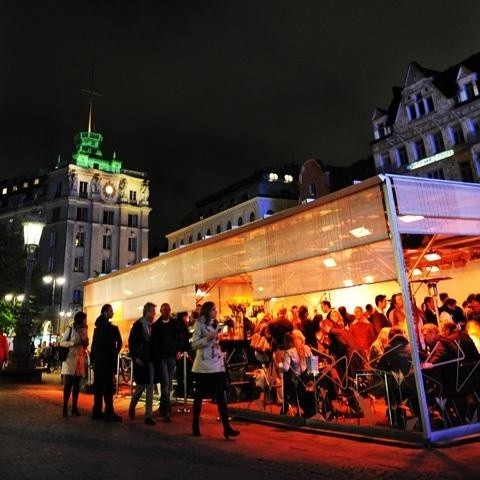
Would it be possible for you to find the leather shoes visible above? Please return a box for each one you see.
[92,409,172,425]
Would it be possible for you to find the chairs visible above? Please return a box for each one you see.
[219,342,480,433]
[83,348,135,400]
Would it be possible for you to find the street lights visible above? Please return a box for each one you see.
[1,216,48,384]
[42,274,66,373]
[5,294,25,323]
[59,310,72,334]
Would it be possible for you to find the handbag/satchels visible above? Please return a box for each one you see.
[57,342,71,362]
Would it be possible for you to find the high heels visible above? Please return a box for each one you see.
[223,427,240,440]
[192,422,201,436]
[63,407,68,417]
[71,407,81,416]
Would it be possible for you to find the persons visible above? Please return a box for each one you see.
[90,305,123,424]
[0,327,9,377]
[59,311,90,418]
